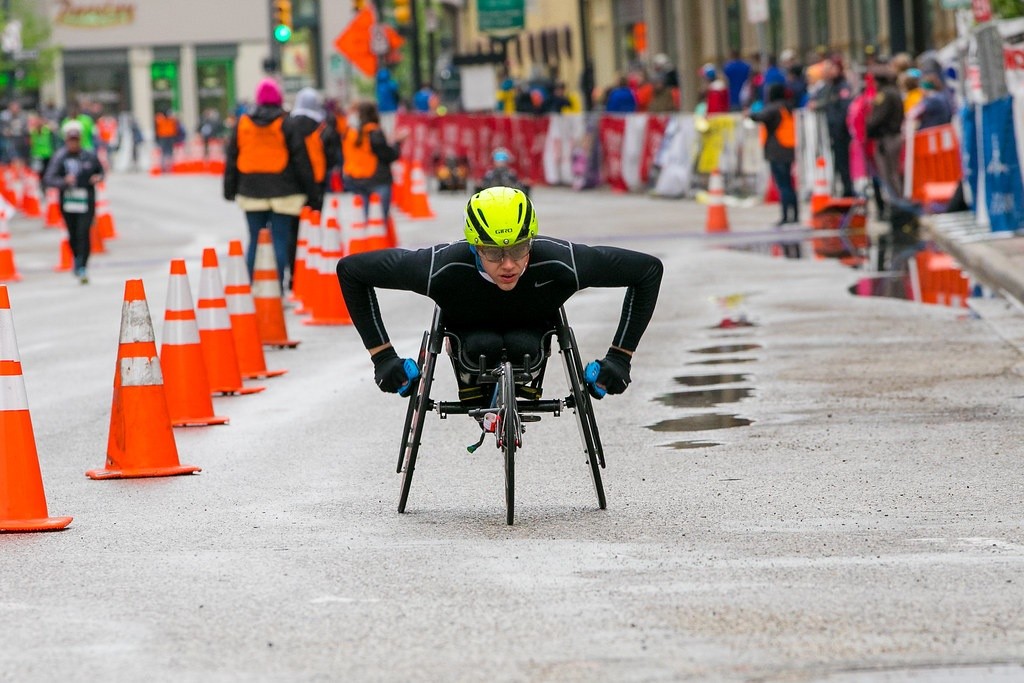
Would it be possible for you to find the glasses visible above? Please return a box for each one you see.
[479,241,531,262]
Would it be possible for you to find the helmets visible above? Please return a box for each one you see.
[463,185,538,247]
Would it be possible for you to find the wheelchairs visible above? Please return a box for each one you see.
[396,287,615,525]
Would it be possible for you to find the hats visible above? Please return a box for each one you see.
[256,78,281,106]
[66,129,80,140]
[290,88,327,123]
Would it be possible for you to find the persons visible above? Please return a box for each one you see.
[224,80,324,308]
[43,128,104,283]
[337,186,664,394]
[1,43,971,290]
[743,83,801,226]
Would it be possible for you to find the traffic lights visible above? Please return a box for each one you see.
[273,0,294,44]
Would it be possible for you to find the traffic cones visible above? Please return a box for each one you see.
[86,277,204,481]
[149,136,228,176]
[704,168,730,233]
[0,283,76,534]
[810,157,831,212]
[0,208,22,282]
[222,240,285,380]
[289,154,434,327]
[194,248,266,396]
[158,259,230,428]
[251,227,302,348]
[0,147,116,273]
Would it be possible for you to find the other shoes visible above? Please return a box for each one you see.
[280,294,298,308]
[79,266,88,284]
[778,219,798,224]
